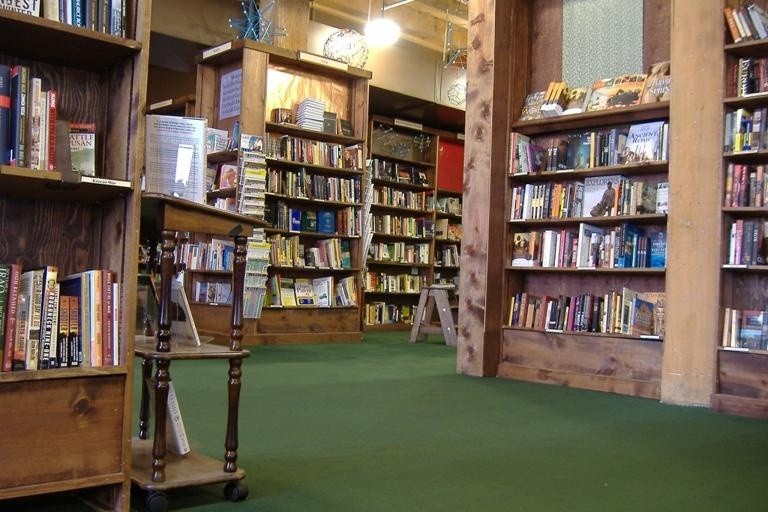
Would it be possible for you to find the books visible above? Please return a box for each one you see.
[517,62,671,121]
[509,122,669,174]
[245,229,359,318]
[150,279,199,348]
[722,0,768,353]
[0,263,120,368]
[204,127,363,237]
[0,0,126,38]
[0,64,95,177]
[273,98,353,136]
[361,159,461,326]
[173,238,232,305]
[511,223,666,269]
[144,374,191,457]
[508,286,665,340]
[509,175,669,220]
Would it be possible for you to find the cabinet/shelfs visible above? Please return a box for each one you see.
[493,35,767,420]
[132,188,271,491]
[0,0,152,511]
